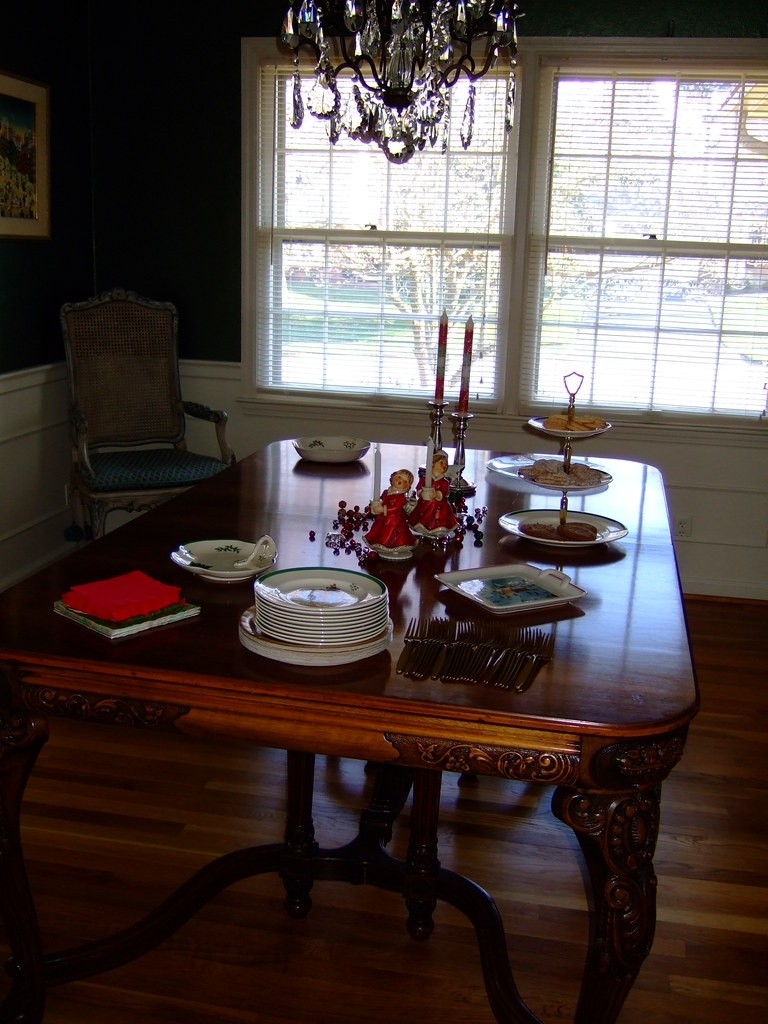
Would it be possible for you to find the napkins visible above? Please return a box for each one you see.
[60,571,180,622]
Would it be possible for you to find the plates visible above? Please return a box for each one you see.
[169,534,278,582]
[291,436,371,463]
[239,567,393,667]
[486,453,598,477]
[433,563,588,613]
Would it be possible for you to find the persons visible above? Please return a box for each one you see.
[363,469,417,555]
[408,449,459,538]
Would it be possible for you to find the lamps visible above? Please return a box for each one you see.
[277,0,518,163]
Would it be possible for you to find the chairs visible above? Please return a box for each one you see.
[61,289,239,542]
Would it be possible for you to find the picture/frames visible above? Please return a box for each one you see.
[0,74,51,239]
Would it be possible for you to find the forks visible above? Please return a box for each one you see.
[395,617,556,694]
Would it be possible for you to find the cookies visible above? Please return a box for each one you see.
[521,522,598,542]
[539,415,606,432]
[518,458,603,487]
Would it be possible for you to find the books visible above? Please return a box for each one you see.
[52,600,201,638]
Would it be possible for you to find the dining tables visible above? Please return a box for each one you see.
[1,434,703,1024]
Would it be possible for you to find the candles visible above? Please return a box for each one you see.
[374,448,380,502]
[424,436,434,488]
[435,307,448,403]
[458,315,474,414]
